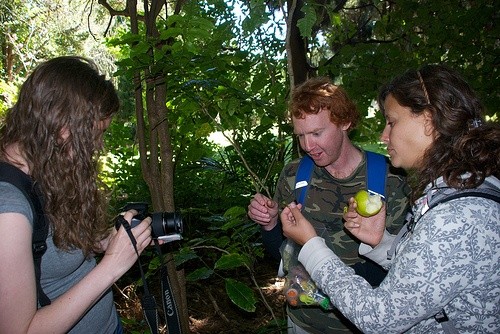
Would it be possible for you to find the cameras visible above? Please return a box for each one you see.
[116,202,184,240]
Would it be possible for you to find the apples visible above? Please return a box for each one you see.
[354,190,383,217]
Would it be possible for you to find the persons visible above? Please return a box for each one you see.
[279,63,500,334]
[0,55,164,334]
[246,76,416,334]
